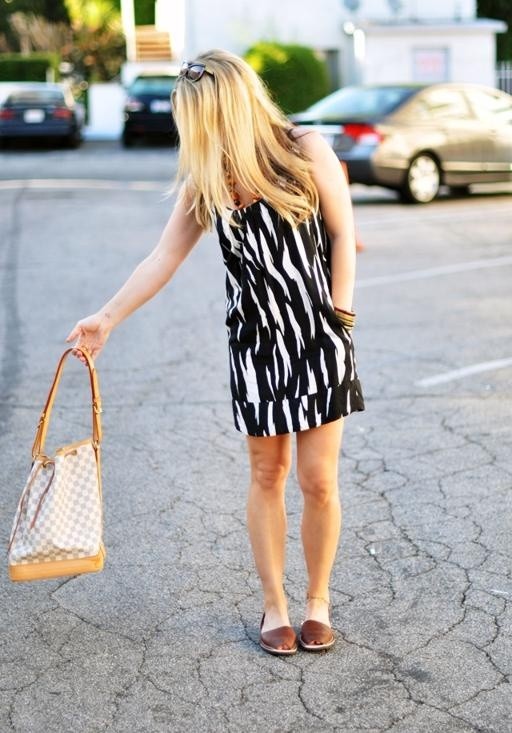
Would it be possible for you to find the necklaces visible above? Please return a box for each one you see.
[221,159,262,209]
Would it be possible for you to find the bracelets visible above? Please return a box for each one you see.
[335,307,356,334]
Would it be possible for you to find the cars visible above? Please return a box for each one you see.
[1,87,85,147]
[121,71,179,145]
[287,82,512,203]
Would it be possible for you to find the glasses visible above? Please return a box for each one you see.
[178,59,215,82]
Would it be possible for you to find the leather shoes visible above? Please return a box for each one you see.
[298,599,335,650]
[259,610,299,655]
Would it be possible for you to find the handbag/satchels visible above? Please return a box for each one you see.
[6,437,107,581]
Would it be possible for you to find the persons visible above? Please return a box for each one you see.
[66,49,364,655]
